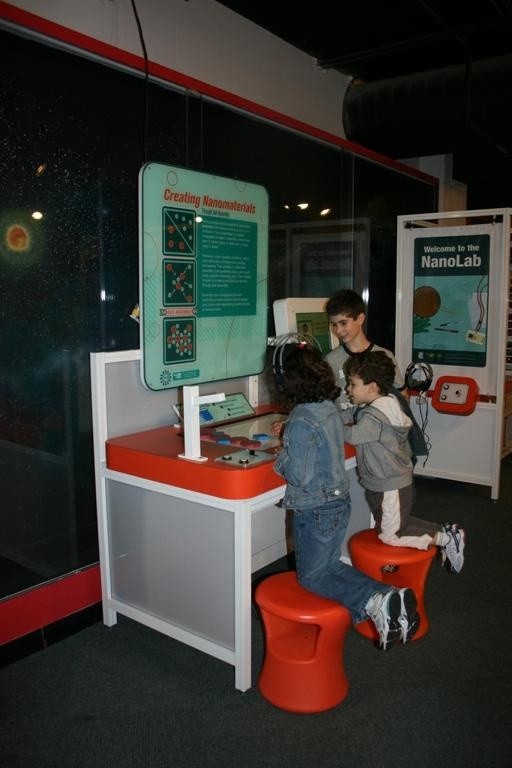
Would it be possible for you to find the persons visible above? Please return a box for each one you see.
[261,342,422,652]
[321,288,411,411]
[337,351,466,574]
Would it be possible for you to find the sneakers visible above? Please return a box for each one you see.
[441,522,460,577]
[367,590,402,652]
[443,529,465,575]
[396,586,421,649]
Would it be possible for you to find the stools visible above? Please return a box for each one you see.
[255,527,438,716]
